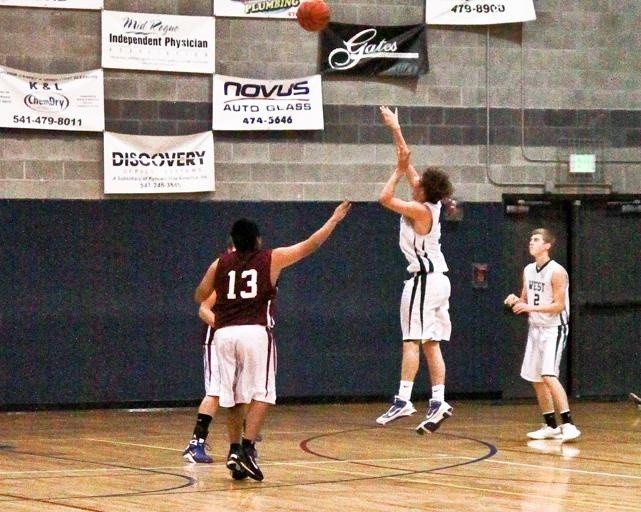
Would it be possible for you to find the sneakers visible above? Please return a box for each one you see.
[375,394,418,425]
[526,423,563,440]
[415,398,454,435]
[560,423,581,443]
[226,445,265,481]
[562,444,580,459]
[527,440,562,457]
[182,434,214,464]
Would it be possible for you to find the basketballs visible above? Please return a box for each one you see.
[297,0,330,32]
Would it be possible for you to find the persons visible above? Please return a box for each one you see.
[501,226,581,445]
[374,101,458,437]
[189,193,354,484]
[180,235,262,465]
